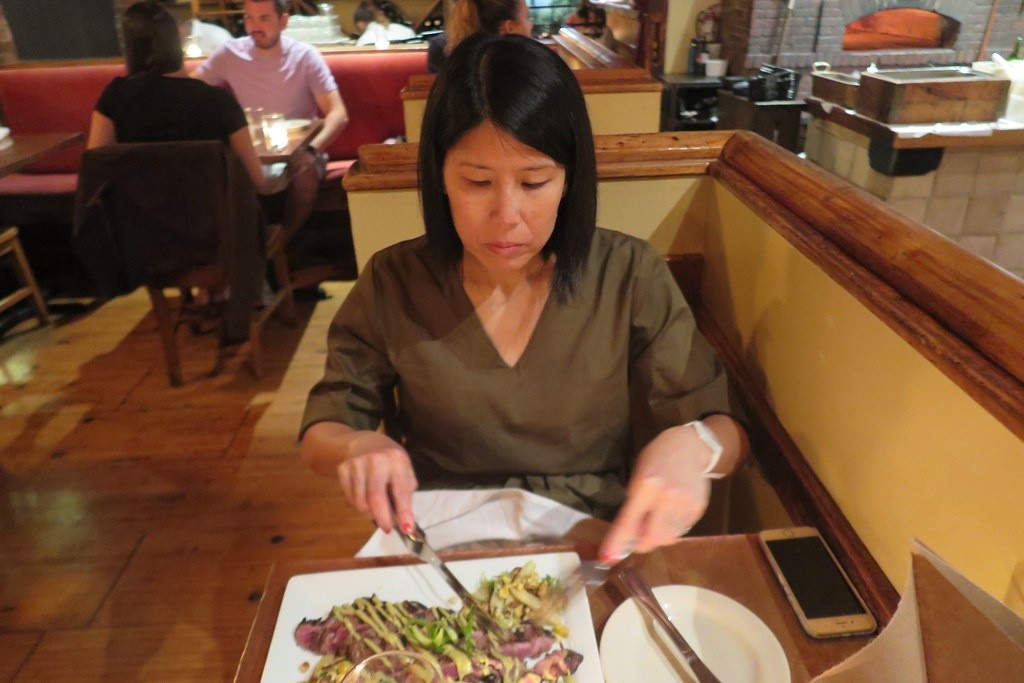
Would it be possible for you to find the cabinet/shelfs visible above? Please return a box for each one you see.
[658,73,726,132]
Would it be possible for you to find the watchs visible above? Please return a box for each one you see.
[304,144,317,155]
[685,420,726,479]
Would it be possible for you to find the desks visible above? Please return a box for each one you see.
[234,533,882,683]
[0,131,84,180]
[255,119,325,334]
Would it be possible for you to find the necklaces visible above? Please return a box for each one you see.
[473,265,545,311]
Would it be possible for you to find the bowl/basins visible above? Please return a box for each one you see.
[705,60,728,77]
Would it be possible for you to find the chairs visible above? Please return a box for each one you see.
[66,140,297,388]
[0,225,52,328]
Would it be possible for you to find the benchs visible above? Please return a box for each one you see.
[0,43,432,269]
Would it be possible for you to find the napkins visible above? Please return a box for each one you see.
[812,539,1023,682]
[355,488,613,558]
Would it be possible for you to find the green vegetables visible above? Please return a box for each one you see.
[405,573,560,651]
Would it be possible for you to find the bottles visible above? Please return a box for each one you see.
[1006,39,1024,61]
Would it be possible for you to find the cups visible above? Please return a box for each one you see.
[261,112,289,151]
[244,106,264,148]
[341,649,442,683]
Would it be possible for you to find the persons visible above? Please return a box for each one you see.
[474,0,534,35]
[85,1,349,332]
[354,4,416,44]
[565,0,613,49]
[297,36,756,564]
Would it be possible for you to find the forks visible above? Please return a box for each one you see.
[534,538,637,623]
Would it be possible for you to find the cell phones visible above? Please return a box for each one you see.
[758,526,877,639]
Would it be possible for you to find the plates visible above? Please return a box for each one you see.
[599,584,792,683]
[286,119,312,131]
[259,551,605,683]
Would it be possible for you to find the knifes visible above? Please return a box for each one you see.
[616,565,722,683]
[389,494,505,636]
